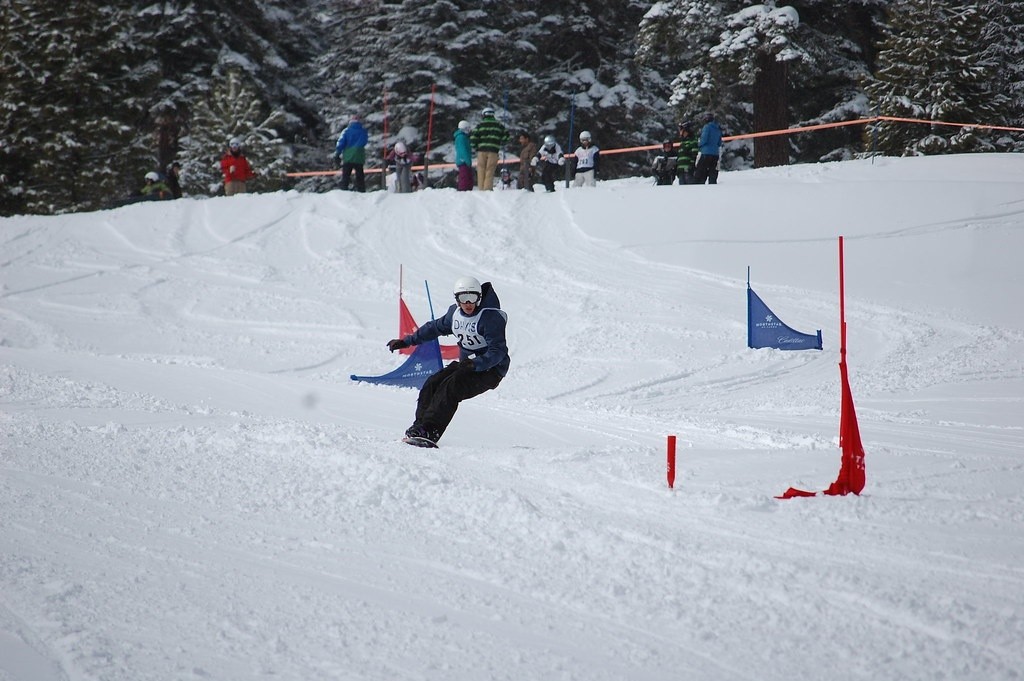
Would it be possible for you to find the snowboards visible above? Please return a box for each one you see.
[401,437,439,448]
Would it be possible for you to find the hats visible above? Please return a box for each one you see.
[679,122,692,132]
[701,112,713,121]
[500,169,510,178]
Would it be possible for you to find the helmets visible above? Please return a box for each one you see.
[453,276,482,307]
[482,107,495,117]
[144,171,159,183]
[580,131,591,143]
[395,142,407,158]
[458,120,470,134]
[545,135,556,147]
[230,138,239,148]
[663,139,673,148]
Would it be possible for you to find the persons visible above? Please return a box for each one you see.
[386,276,510,448]
[126,161,182,201]
[570,131,601,188]
[220,138,257,197]
[497,168,517,190]
[385,142,425,193]
[453,120,474,191]
[530,135,565,193]
[651,112,723,185]
[470,107,509,191]
[335,113,369,193]
[516,132,540,192]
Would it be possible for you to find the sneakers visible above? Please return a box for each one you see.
[405,424,441,443]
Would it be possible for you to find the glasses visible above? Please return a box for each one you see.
[455,291,481,304]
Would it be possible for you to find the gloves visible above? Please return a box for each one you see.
[457,358,476,373]
[387,339,411,353]
